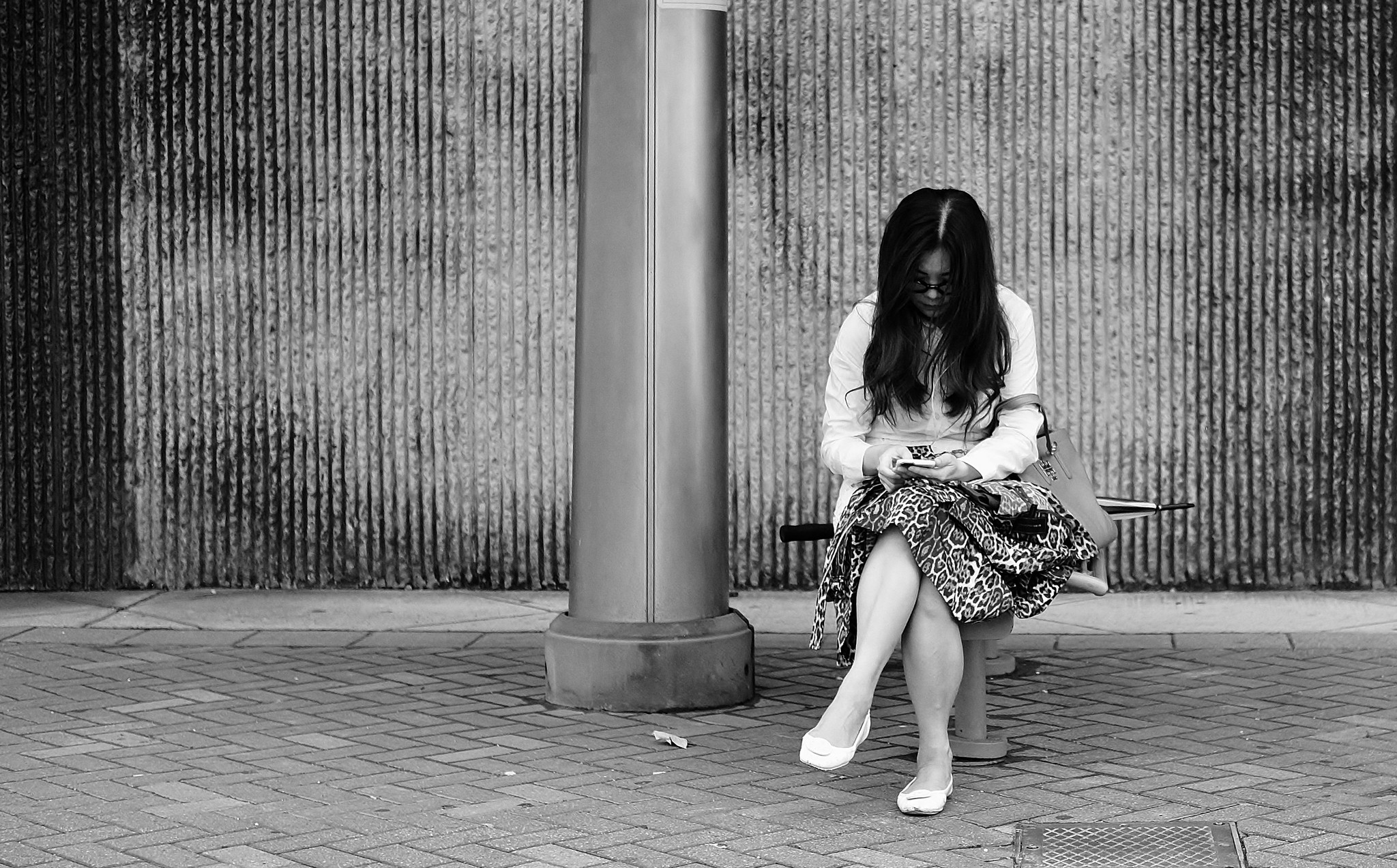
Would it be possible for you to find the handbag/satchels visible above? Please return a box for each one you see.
[997,393,1120,552]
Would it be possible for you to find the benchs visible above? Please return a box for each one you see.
[949,547,1110,758]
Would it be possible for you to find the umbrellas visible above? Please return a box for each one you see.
[780,497,1195,543]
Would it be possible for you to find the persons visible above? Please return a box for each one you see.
[798,185,1100,817]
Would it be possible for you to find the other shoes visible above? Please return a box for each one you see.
[896,772,954,817]
[799,709,872,771]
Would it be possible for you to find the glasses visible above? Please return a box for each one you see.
[909,278,957,295]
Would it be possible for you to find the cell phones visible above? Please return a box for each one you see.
[895,458,935,469]
